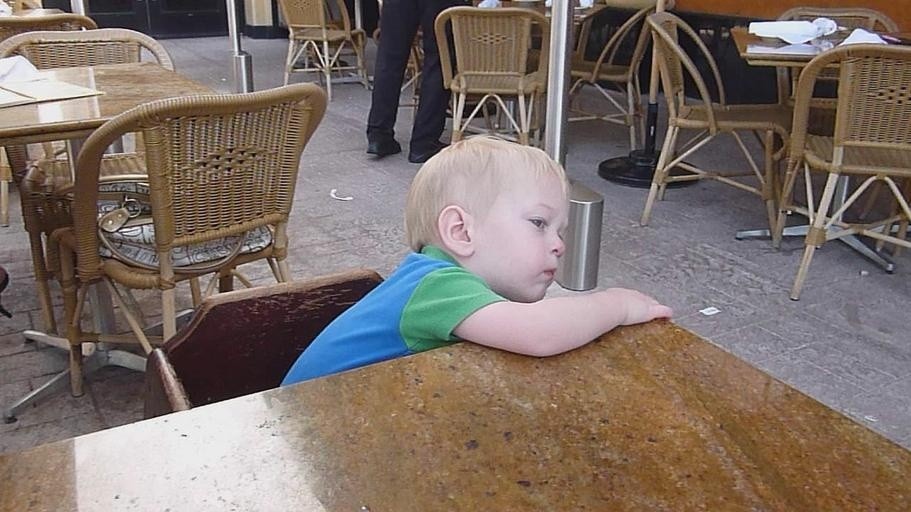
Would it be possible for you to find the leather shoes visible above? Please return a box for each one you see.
[366,139,401,155]
[409,141,449,163]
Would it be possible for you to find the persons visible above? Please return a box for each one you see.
[365,0,473,165]
[280,133,673,387]
